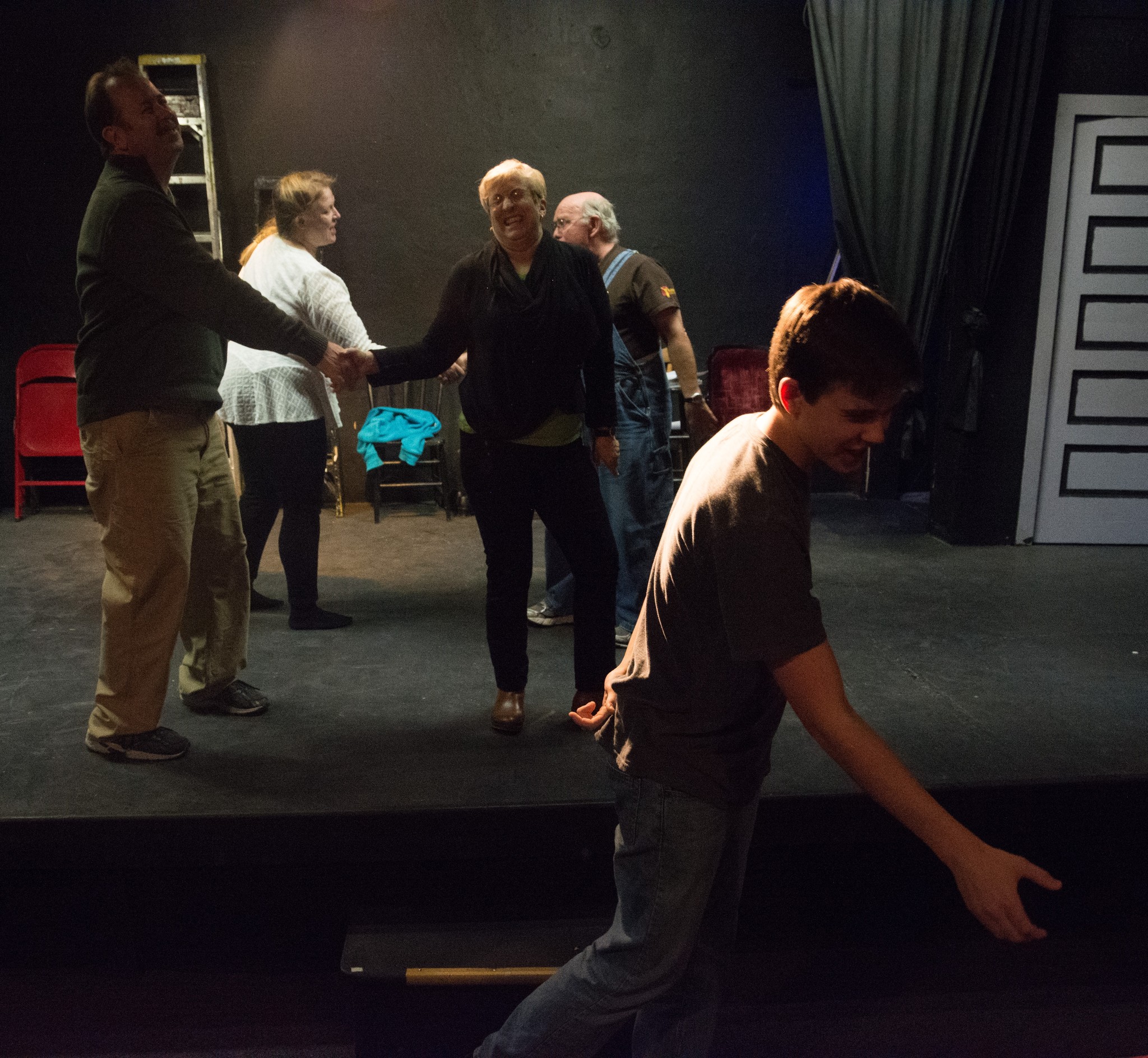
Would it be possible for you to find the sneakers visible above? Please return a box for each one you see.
[288,604,353,629]
[527,599,574,626]
[85,726,192,762]
[614,626,633,648]
[250,590,284,609]
[210,679,269,715]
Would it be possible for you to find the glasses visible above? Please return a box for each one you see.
[486,188,534,207]
[553,216,587,229]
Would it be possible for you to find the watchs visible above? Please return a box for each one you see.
[684,392,704,406]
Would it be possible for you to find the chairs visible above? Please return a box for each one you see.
[316,372,343,518]
[363,374,452,525]
[12,344,84,523]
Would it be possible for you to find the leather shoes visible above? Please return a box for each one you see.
[490,689,525,733]
[571,690,604,718]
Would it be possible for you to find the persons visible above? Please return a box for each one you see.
[470,276,1064,1057]
[440,191,718,647]
[75,65,361,761]
[239,176,277,268]
[211,172,465,631]
